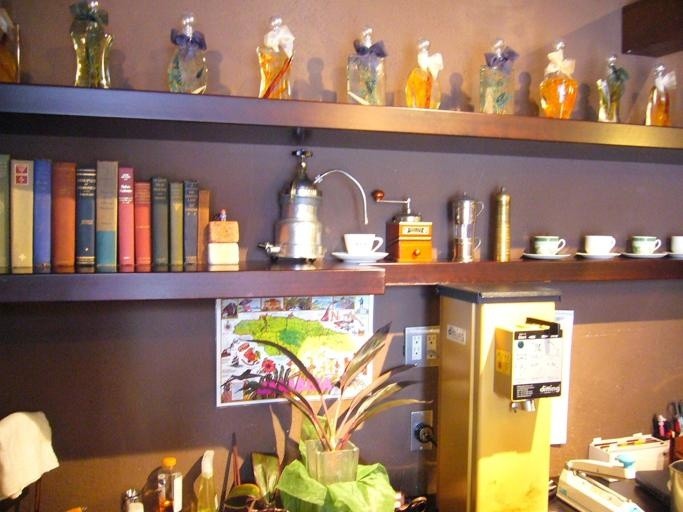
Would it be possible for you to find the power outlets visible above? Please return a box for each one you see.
[411,410,433,452]
[405,326,441,368]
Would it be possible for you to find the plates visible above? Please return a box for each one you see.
[621,250,667,259]
[521,252,573,259]
[576,252,621,260]
[331,251,386,263]
[667,251,682,260]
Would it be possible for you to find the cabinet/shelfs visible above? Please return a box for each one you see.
[0,85,683,301]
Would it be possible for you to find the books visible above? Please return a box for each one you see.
[0,153,210,274]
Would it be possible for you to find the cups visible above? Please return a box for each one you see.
[583,234,616,253]
[670,235,683,252]
[629,235,661,254]
[532,235,566,254]
[343,233,384,254]
[669,461,682,511]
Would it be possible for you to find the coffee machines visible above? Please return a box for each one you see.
[373,189,434,264]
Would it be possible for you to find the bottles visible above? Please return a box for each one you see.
[537,41,579,120]
[643,65,678,127]
[257,16,295,100]
[449,191,486,263]
[167,12,209,95]
[195,449,221,511]
[478,37,520,115]
[346,26,388,104]
[123,489,144,512]
[68,1,116,87]
[405,39,444,111]
[153,457,182,512]
[593,54,629,121]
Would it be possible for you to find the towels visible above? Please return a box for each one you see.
[0,411,59,500]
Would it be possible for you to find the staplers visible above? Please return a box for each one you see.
[556,459,645,512]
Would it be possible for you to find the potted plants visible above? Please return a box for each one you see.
[219,319,432,512]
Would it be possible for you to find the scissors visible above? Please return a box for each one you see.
[667,400,683,428]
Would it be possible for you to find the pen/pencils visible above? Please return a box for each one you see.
[652,414,681,446]
